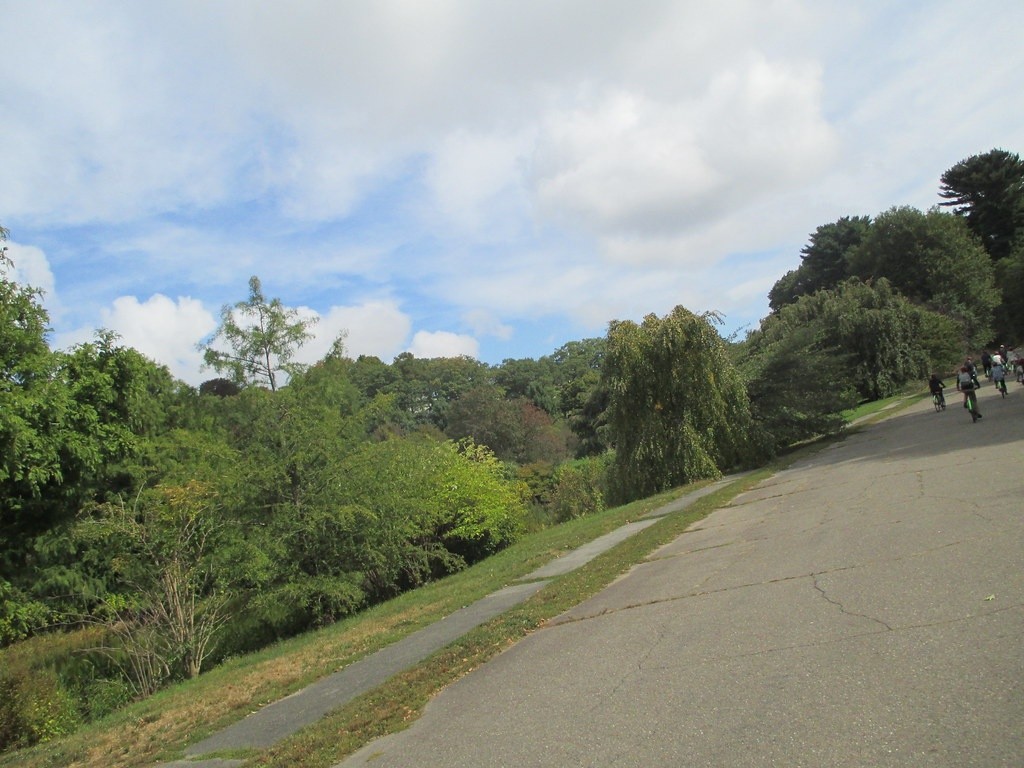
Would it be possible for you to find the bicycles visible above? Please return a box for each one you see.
[932,387,946,413]
[994,374,1008,399]
[1001,355,1008,376]
[984,363,991,379]
[958,387,979,423]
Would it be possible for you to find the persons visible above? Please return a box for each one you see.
[987,362,1009,395]
[982,345,1024,383]
[964,356,981,388]
[957,367,982,417]
[929,374,946,406]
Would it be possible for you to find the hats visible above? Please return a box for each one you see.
[1000,345,1005,347]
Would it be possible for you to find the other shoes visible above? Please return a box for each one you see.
[941,405,946,407]
[996,387,998,389]
[977,413,982,418]
[1005,393,1009,395]
[963,402,967,409]
